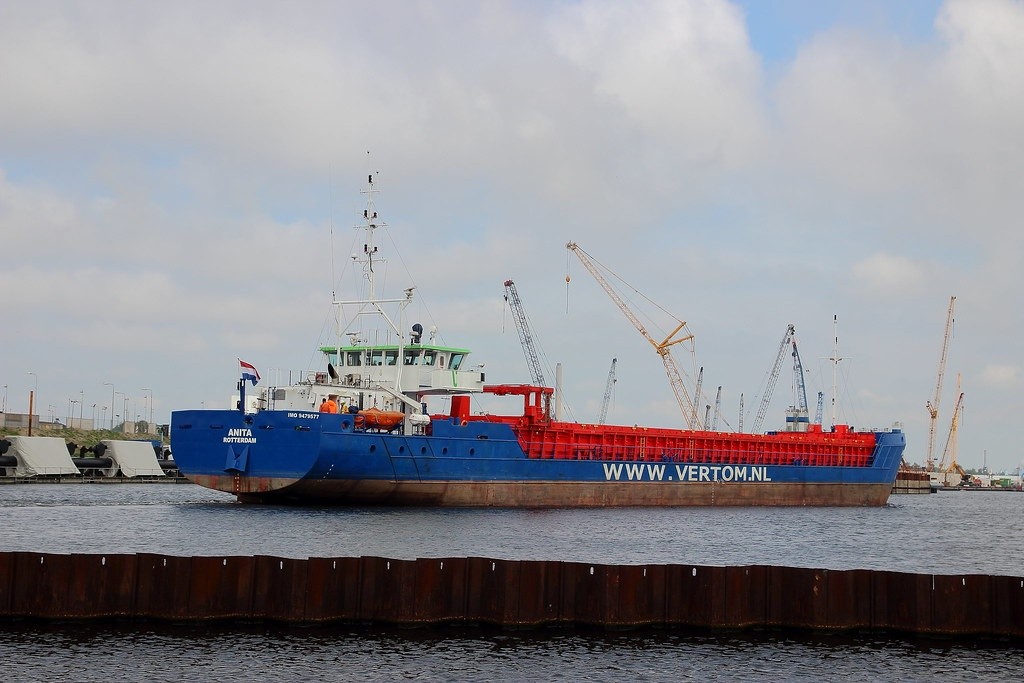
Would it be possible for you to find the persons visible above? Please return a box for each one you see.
[319,397,347,414]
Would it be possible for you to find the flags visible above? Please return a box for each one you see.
[240,360,261,387]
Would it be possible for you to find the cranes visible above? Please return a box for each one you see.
[501,278,556,420]
[565,240,833,433]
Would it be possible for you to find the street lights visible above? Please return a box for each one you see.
[3,384,8,429]
[142,388,153,423]
[115,391,126,422]
[67,389,106,430]
[28,372,37,415]
[105,382,115,431]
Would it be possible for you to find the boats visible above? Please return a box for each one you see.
[168,164,907,509]
[357,407,407,430]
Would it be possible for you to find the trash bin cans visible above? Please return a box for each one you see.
[1000,478,1011,487]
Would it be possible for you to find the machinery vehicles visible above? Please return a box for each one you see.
[922,295,984,487]
